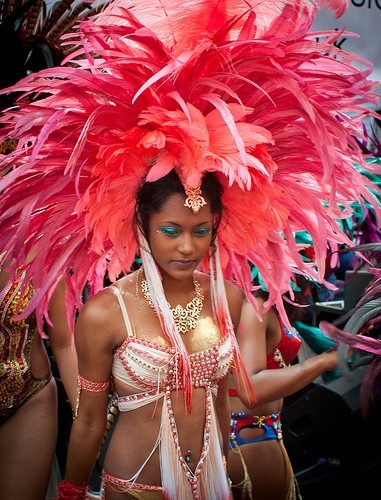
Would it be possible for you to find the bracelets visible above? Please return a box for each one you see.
[54,480,87,500]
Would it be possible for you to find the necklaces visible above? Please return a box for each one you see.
[137,266,206,334]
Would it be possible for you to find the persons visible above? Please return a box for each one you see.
[0,219,87,500]
[48,141,247,500]
[229,271,350,500]
